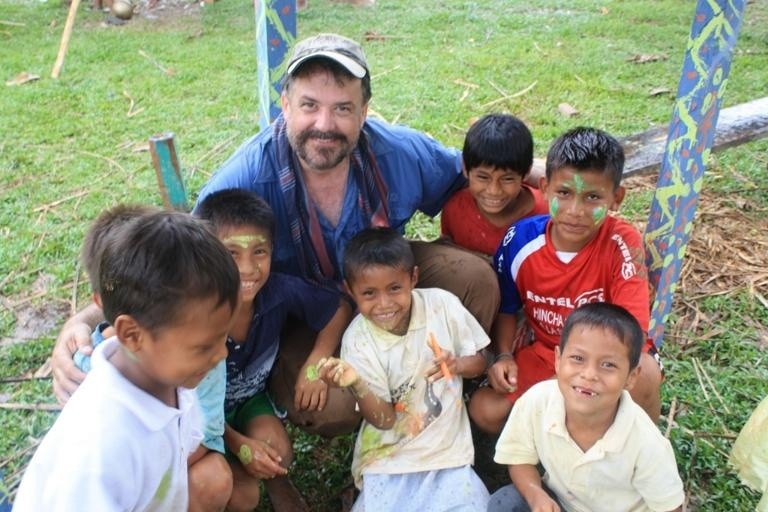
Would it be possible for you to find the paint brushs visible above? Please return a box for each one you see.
[429,332,462,408]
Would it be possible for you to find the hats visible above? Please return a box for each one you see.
[286,32,369,79]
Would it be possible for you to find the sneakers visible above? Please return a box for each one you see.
[264,475,310,512]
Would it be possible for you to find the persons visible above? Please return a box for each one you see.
[314,228,489,509]
[13,207,242,511]
[53,31,545,437]
[442,114,687,432]
[486,302,685,511]
[76,189,353,512]
